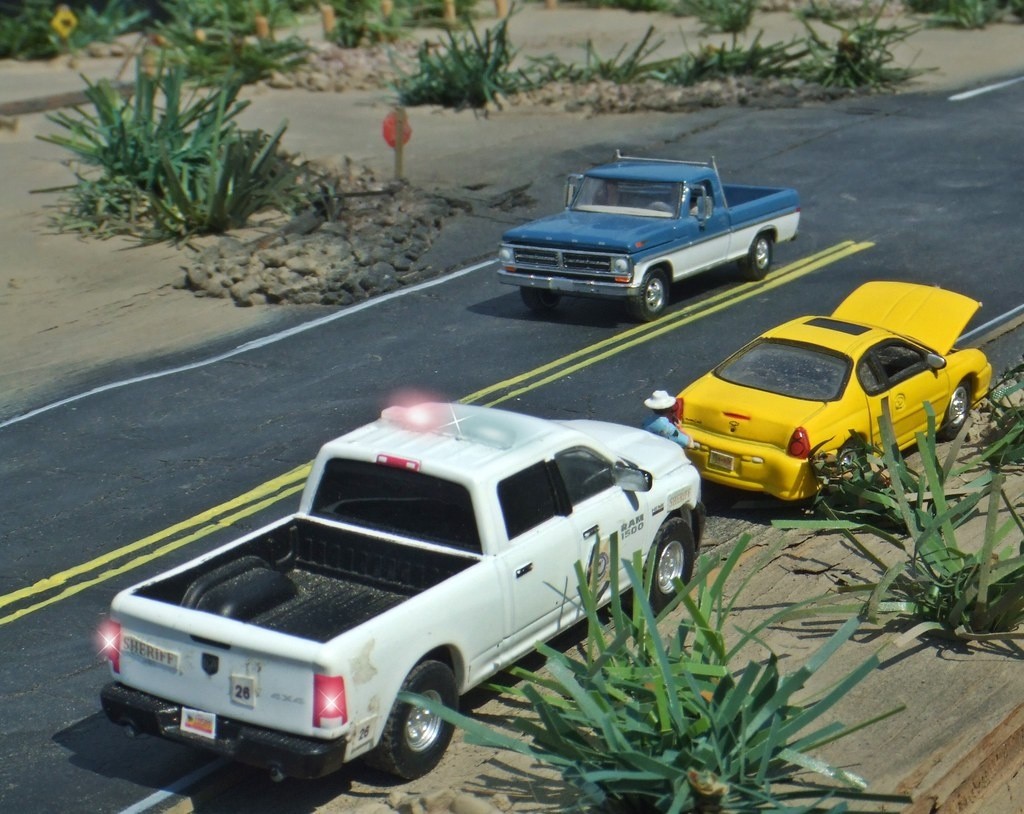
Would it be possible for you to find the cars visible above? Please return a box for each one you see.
[672,282,995,502]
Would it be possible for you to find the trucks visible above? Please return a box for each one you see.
[99,400,705,784]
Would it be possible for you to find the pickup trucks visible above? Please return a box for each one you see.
[498,149,803,323]
